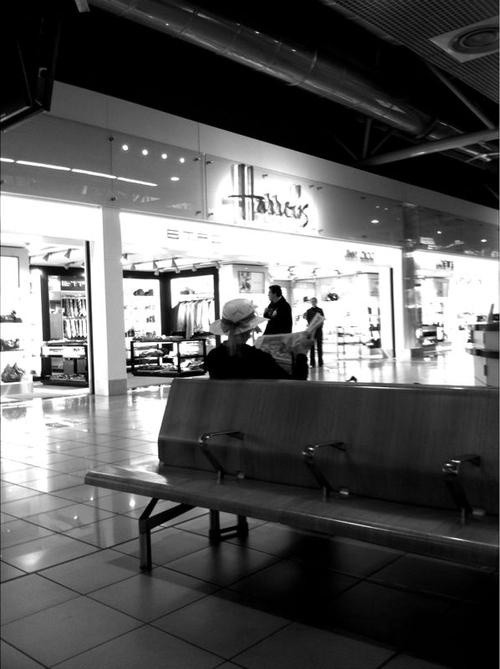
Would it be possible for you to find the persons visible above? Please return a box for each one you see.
[204,299,312,380]
[263,284,292,334]
[304,297,324,368]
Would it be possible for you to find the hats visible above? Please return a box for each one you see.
[208,299,271,337]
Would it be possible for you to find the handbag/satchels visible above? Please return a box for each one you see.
[1,362,25,383]
